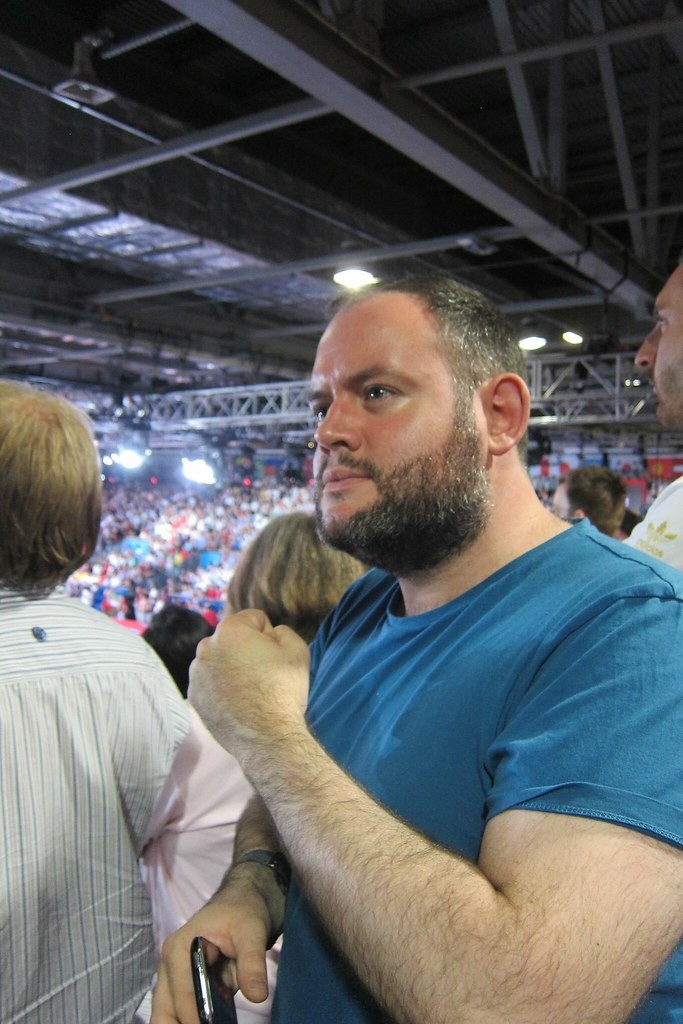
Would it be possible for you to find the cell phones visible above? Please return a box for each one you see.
[189,935,239,1024]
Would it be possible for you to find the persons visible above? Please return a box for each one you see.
[0,262,683,1024]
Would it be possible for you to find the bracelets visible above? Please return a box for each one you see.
[232,848,291,901]
